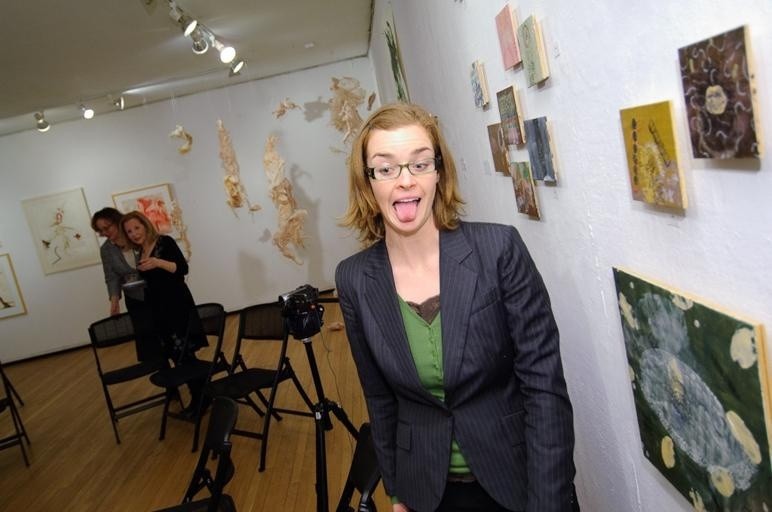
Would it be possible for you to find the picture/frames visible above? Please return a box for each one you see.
[0,250,28,320]
[19,186,103,277]
[110,182,184,244]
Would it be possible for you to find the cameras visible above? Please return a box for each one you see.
[277,285,321,326]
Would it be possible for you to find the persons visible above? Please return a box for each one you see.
[336,103,581,512]
[90,207,159,363]
[124,211,210,417]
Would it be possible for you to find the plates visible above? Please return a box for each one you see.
[121,281,145,288]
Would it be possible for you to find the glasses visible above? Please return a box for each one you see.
[365,155,441,180]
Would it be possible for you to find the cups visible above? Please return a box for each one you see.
[124,272,137,282]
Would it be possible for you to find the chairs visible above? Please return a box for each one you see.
[161,400,239,511]
[205,303,320,471]
[85,310,186,445]
[150,303,265,453]
[0,359,34,469]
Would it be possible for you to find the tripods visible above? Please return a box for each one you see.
[301,341,360,512]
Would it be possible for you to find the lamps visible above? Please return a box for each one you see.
[33,92,126,133]
[167,0,247,75]
